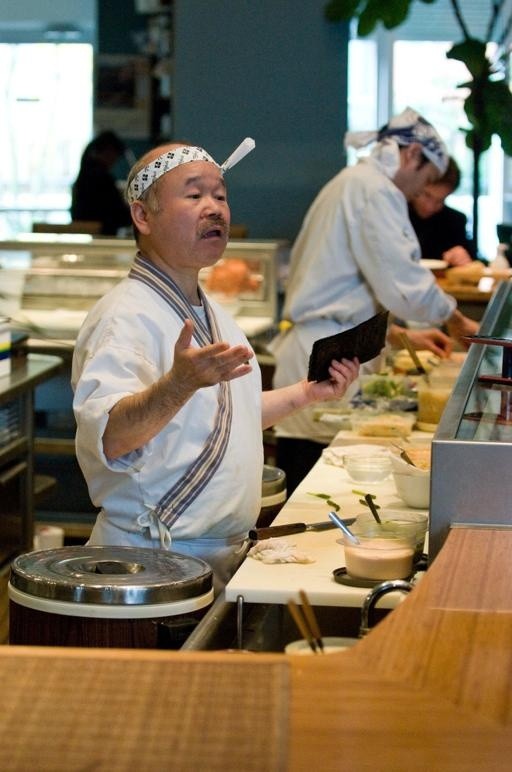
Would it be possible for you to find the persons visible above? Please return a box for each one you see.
[408,156,479,265]
[71,143,361,646]
[272,105,480,499]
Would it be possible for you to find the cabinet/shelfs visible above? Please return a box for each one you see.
[0,233,293,456]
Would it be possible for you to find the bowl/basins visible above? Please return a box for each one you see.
[337,533,420,580]
[393,472,429,509]
[350,412,416,437]
[285,638,359,660]
[343,454,393,482]
[357,511,428,564]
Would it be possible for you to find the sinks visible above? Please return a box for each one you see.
[186,600,393,656]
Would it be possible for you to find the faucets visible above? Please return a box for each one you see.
[359,579,415,638]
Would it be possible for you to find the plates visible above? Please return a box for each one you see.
[333,567,412,589]
[416,554,429,570]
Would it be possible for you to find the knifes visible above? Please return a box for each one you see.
[249,518,356,540]
